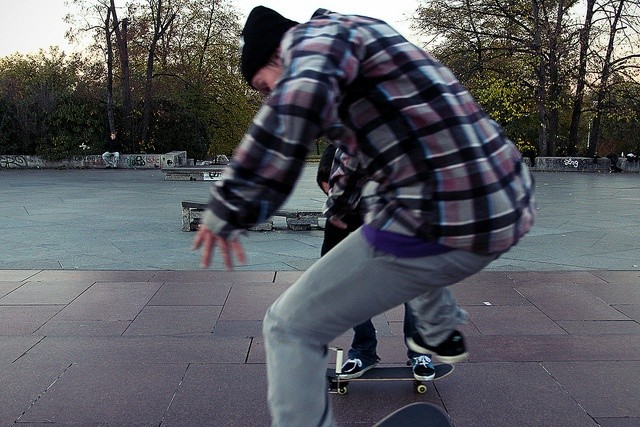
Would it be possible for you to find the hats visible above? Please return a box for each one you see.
[241,5,300,89]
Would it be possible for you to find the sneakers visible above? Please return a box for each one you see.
[338,357,377,380]
[406,329,469,364]
[411,355,436,381]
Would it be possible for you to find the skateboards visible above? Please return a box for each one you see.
[372,401,454,427]
[326,362,454,394]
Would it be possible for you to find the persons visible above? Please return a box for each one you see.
[189,6,536,425]
[316,142,438,382]
